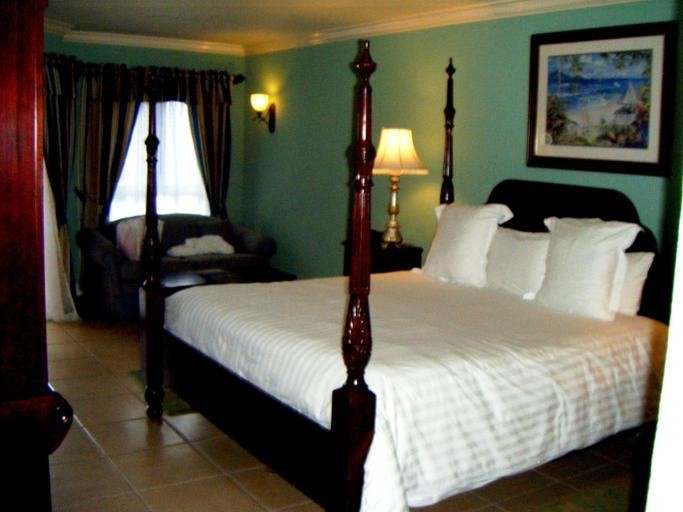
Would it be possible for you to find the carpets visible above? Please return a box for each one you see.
[129,368,191,420]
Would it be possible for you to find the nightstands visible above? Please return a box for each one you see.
[339,232,421,277]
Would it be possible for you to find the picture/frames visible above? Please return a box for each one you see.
[521,16,670,179]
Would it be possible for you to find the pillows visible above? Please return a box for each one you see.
[415,201,510,288]
[480,222,555,300]
[524,213,641,323]
[617,247,652,316]
[115,216,163,263]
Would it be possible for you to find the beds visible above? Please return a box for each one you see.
[139,41,670,512]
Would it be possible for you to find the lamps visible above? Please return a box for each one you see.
[371,126,432,252]
[247,91,278,132]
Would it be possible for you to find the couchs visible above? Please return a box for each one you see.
[75,217,299,324]
